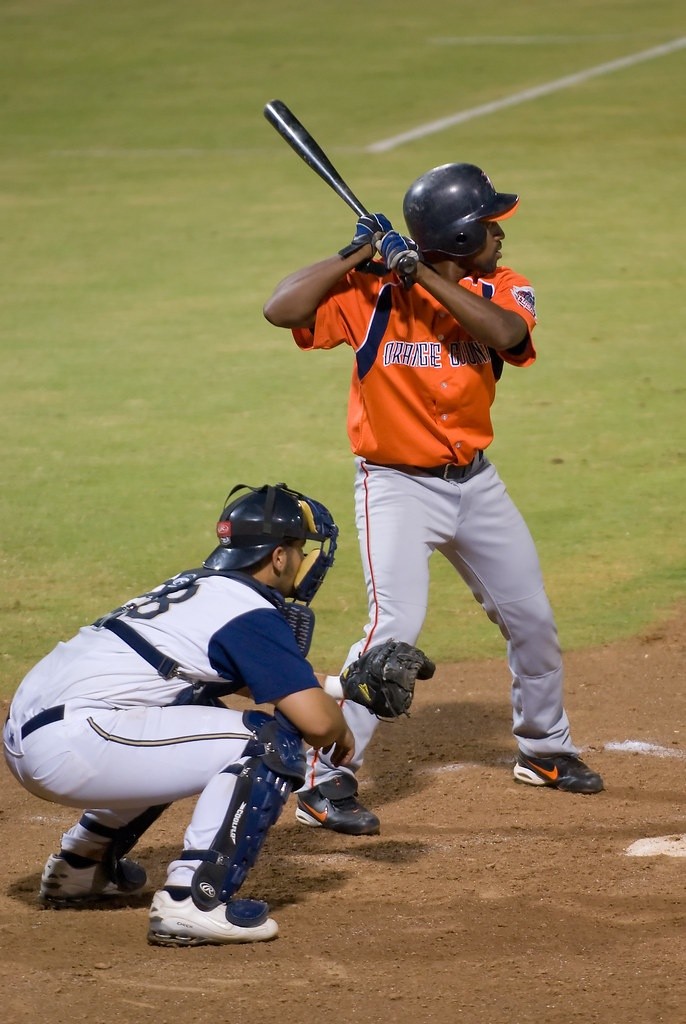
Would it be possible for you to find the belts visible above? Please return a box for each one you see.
[366,450,483,481]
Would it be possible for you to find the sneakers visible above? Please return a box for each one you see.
[296,774,380,835]
[513,748,602,793]
[38,853,152,906]
[148,889,279,946]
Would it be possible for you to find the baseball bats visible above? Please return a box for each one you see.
[264,100,418,276]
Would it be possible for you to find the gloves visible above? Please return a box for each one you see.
[375,231,423,280]
[352,213,394,256]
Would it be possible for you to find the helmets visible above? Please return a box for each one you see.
[403,163,520,257]
[202,488,308,571]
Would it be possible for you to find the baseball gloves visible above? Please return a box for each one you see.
[340,637,437,725]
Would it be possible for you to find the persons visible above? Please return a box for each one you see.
[1,482,435,949]
[262,163,604,835]
